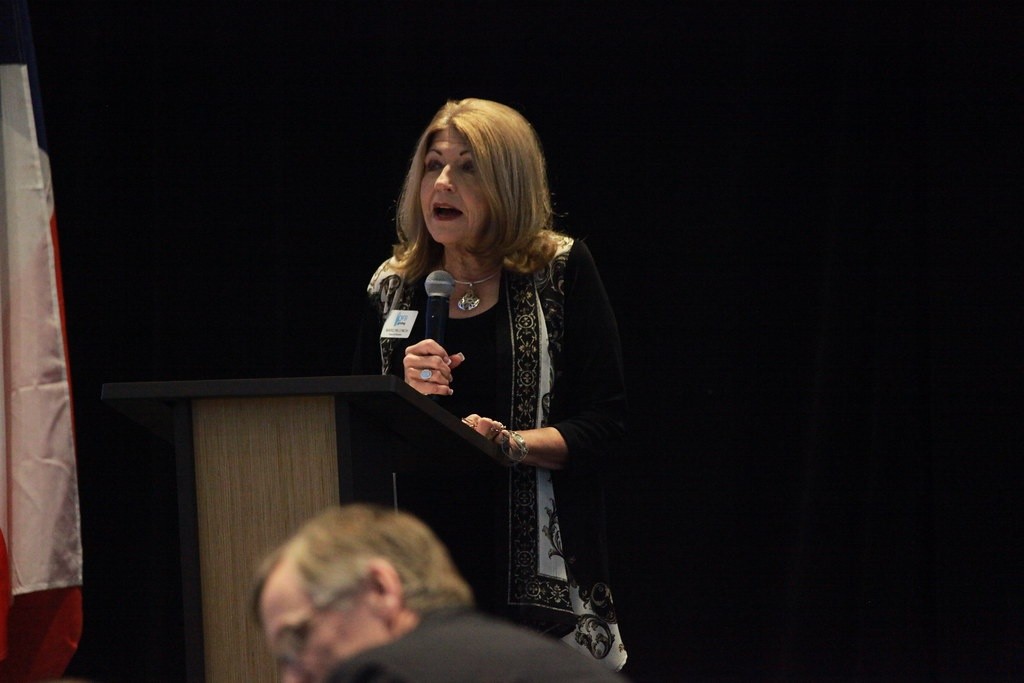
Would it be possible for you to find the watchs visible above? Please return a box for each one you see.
[508,429,526,460]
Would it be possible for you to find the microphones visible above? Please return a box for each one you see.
[425,270,455,411]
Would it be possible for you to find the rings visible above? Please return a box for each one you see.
[491,421,503,432]
[421,369,432,382]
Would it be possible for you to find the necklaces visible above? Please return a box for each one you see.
[439,260,500,311]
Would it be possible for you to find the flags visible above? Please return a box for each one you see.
[0,1,86,683]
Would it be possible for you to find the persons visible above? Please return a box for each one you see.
[352,97,628,673]
[250,504,624,683]
